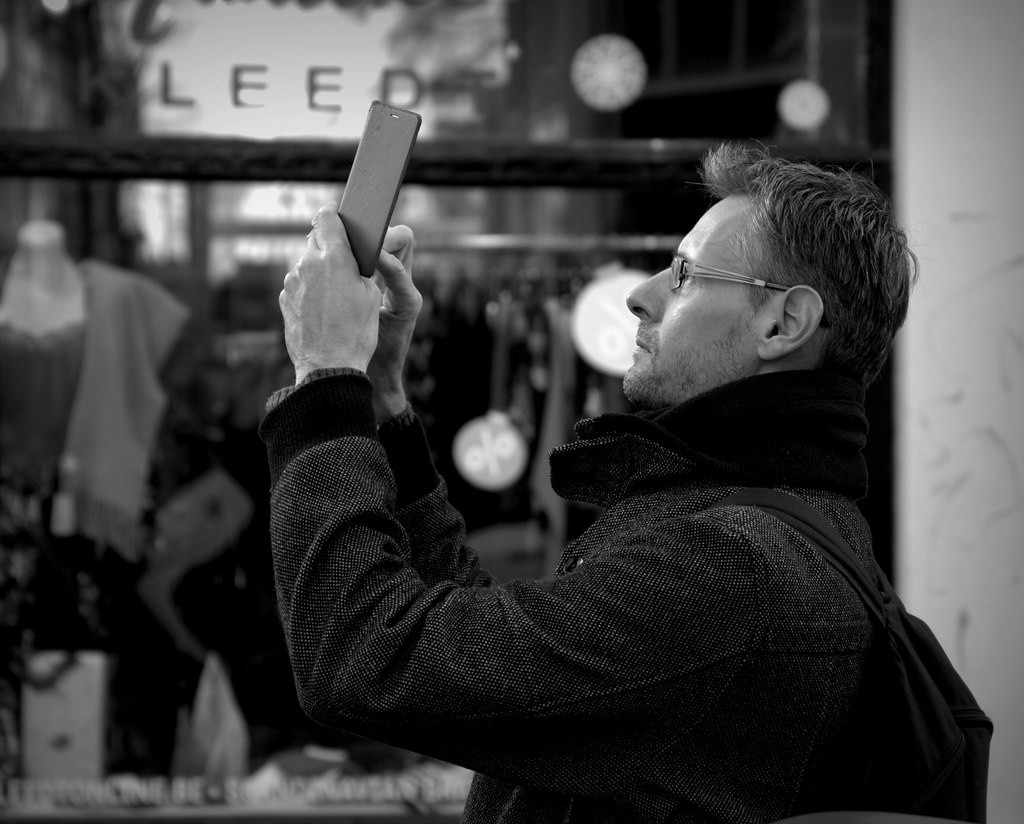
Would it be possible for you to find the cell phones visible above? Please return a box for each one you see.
[338,100,421,278]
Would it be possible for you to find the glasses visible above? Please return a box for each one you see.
[669,255,829,328]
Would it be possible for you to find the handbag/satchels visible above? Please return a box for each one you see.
[16,530,135,686]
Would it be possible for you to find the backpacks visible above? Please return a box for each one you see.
[707,490,994,824]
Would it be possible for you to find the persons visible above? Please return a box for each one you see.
[0,216,140,546]
[260,133,921,824]
